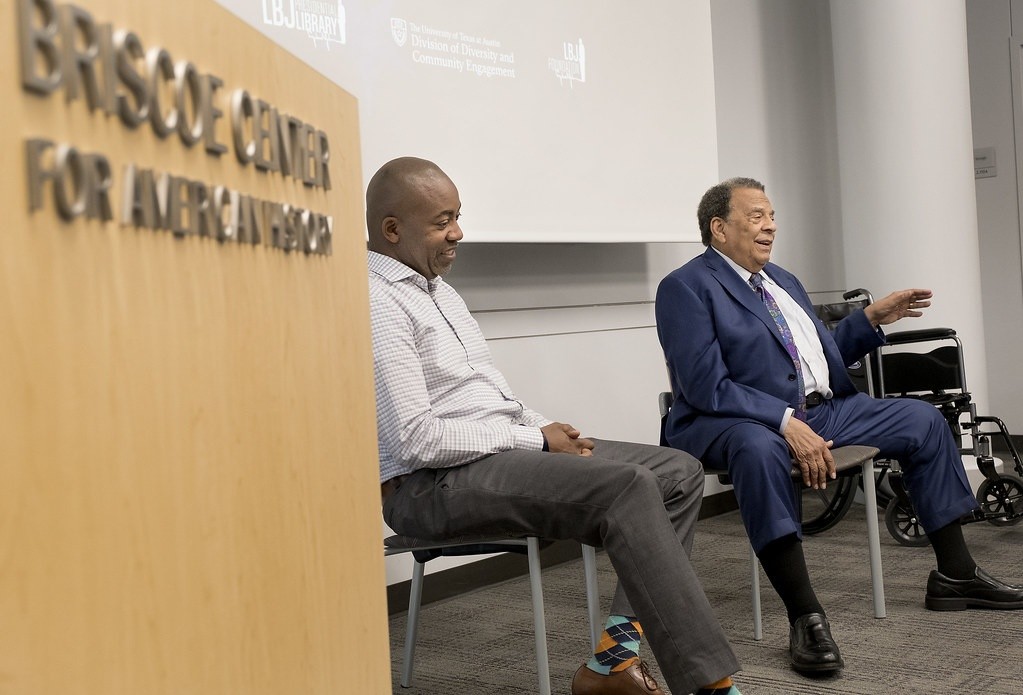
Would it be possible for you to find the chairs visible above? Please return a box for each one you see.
[657,393,888,641]
[383,534,603,694]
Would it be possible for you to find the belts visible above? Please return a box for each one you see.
[805,392,823,408]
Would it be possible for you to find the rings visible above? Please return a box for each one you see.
[909,302,914,309]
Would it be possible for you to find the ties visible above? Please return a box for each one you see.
[749,272,806,422]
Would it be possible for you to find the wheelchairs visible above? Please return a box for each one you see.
[793,288,1023,546]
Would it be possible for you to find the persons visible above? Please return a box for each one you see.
[366,156,745,695]
[654,175,1023,675]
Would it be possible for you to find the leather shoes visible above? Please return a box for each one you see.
[790,612,844,672]
[572,658,666,695]
[925,566,1023,611]
